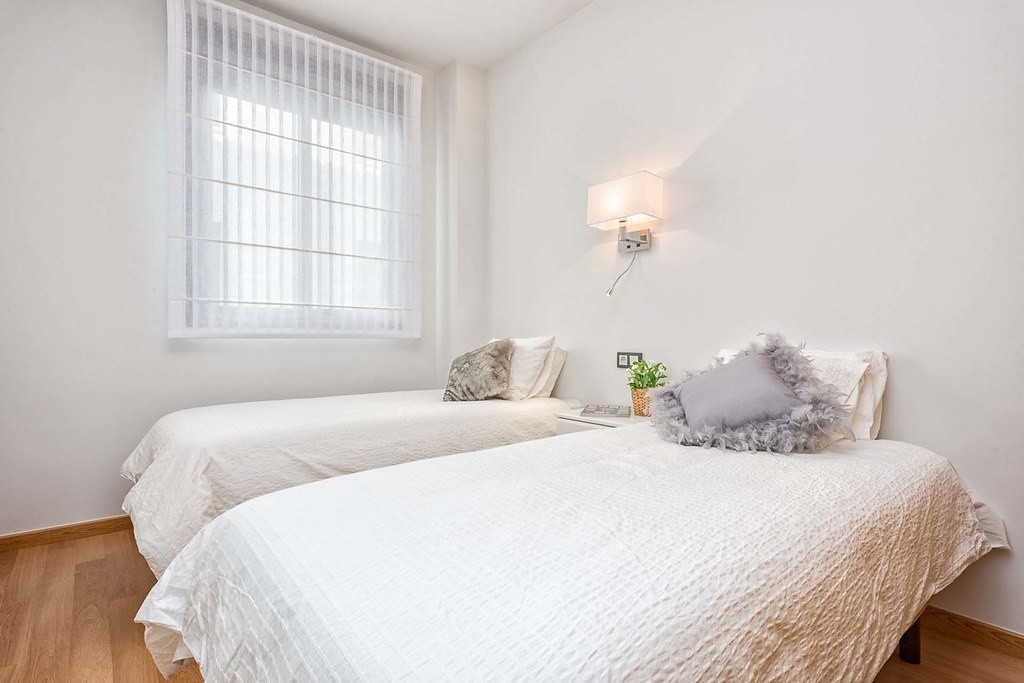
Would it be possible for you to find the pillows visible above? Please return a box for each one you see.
[712,345,890,440]
[528,345,568,399]
[642,327,855,457]
[442,337,513,403]
[485,336,555,401]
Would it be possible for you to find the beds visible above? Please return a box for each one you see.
[135,435,1012,683]
[119,384,581,581]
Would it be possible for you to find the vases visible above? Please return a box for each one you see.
[623,357,670,418]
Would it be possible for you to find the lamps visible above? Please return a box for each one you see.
[584,169,665,252]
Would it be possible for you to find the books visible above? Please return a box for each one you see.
[580,403,632,418]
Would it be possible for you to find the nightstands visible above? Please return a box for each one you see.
[554,406,646,436]
